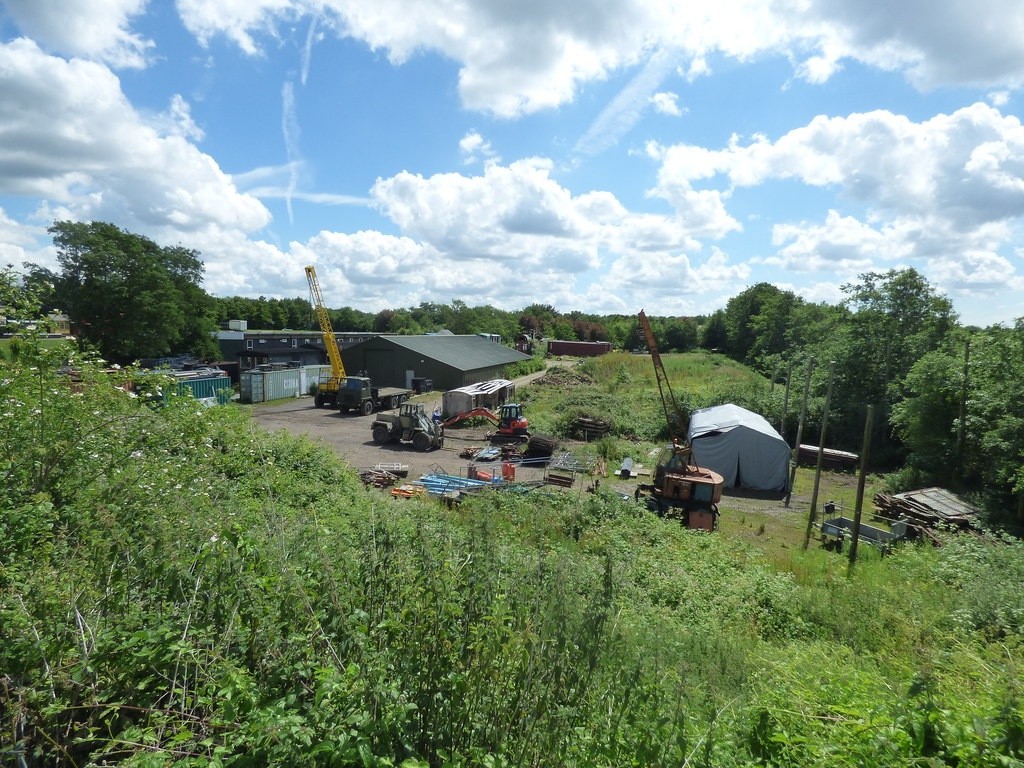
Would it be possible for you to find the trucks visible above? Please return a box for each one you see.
[335,375,414,415]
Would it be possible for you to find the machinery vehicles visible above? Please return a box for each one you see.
[444,403,529,442]
[638,308,725,523]
[370,401,444,449]
[305,264,347,410]
